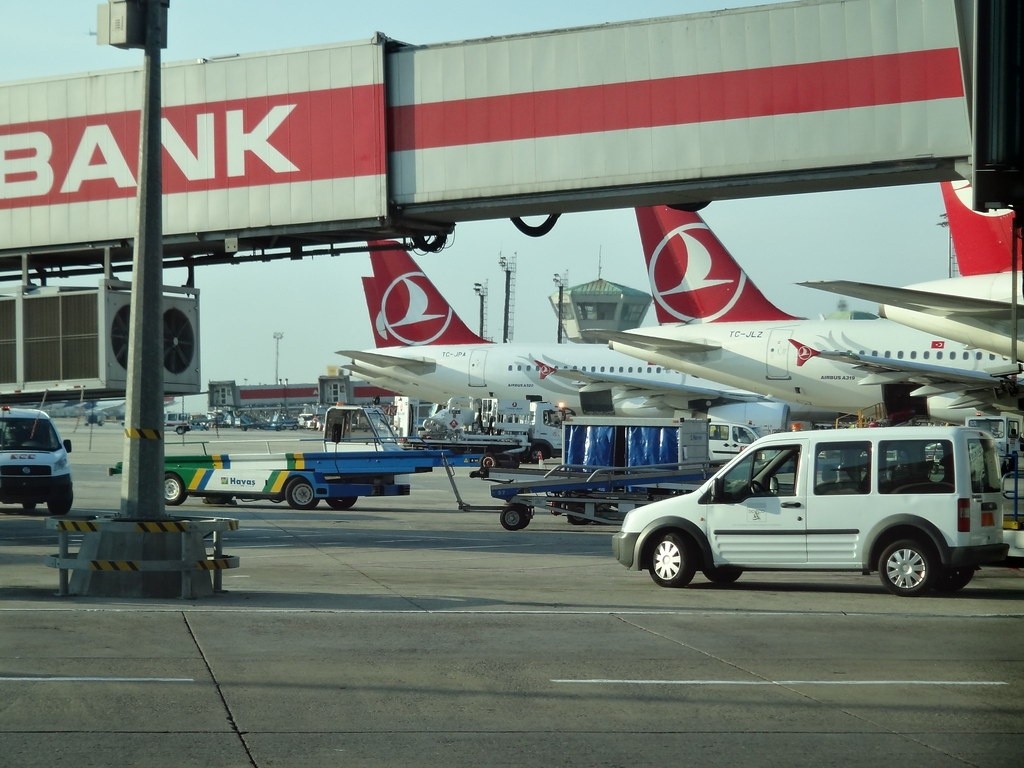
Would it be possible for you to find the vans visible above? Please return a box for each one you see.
[0,404,75,518]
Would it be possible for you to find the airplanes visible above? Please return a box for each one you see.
[335,175,1024,470]
[82,405,110,426]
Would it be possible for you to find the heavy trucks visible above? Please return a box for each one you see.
[397,397,578,464]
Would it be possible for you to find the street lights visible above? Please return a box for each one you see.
[472,278,487,339]
[273,331,283,384]
[552,269,569,343]
[497,250,518,344]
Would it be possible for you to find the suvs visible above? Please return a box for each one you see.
[163,408,326,435]
[610,425,1005,598]
